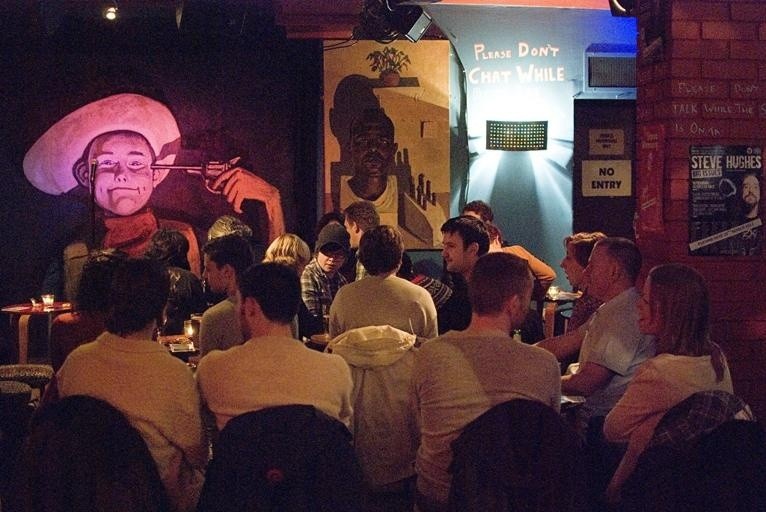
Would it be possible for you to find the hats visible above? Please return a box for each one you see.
[316,223,352,257]
[22,93,182,197]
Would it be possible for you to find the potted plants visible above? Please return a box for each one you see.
[366,47,410,86]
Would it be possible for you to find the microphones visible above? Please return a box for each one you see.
[89,158,97,183]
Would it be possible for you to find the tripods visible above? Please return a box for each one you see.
[69,182,114,266]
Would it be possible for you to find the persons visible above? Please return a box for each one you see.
[40,130,286,306]
[604,264,733,497]
[410,252,561,510]
[730,170,766,255]
[530,231,657,447]
[332,109,398,228]
[49,202,556,372]
[55,258,206,499]
[192,262,354,437]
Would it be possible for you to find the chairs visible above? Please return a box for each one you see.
[0,364,54,400]
[197,405,363,512]
[448,398,585,512]
[0,381,31,437]
[623,390,754,512]
[31,396,168,512]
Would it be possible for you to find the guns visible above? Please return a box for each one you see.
[152,157,260,230]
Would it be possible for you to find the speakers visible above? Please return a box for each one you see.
[582,52,637,93]
[608,0,668,16]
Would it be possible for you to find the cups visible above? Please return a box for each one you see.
[41,294,55,309]
[548,285,564,298]
[182,312,202,348]
[321,312,334,339]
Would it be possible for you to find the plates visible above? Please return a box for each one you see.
[169,343,196,354]
